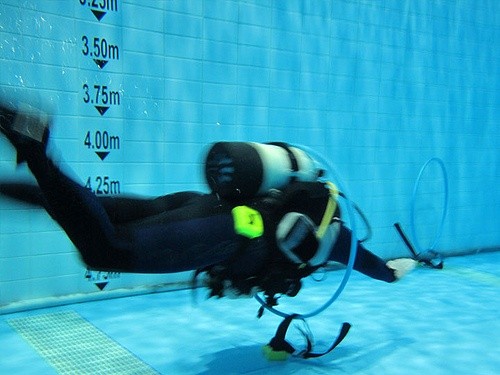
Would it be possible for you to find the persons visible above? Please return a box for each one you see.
[1,97,420,301]
